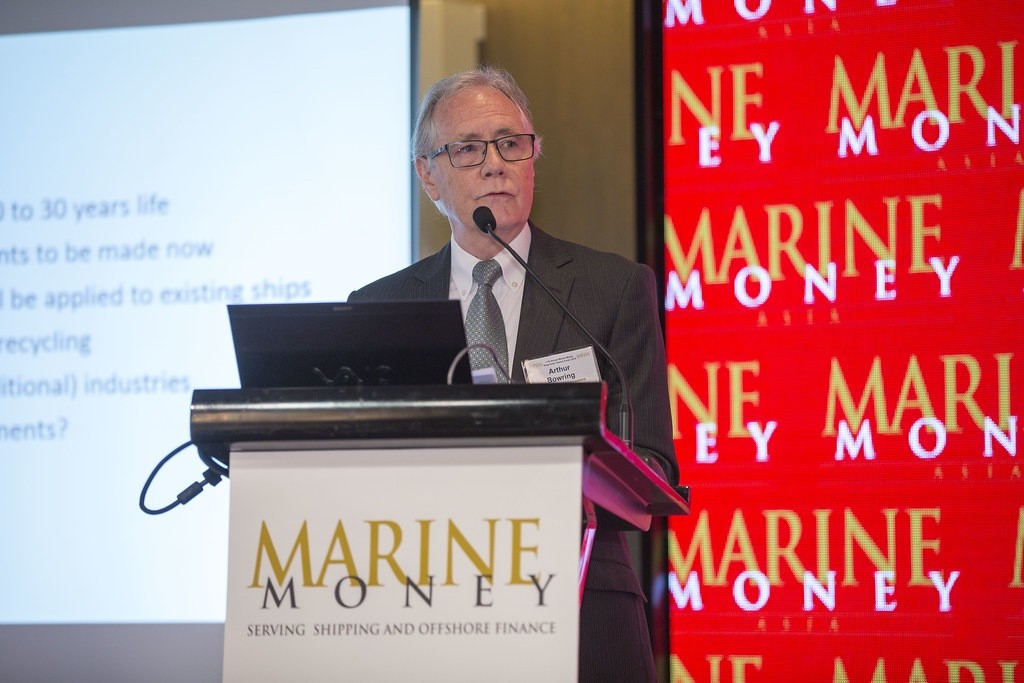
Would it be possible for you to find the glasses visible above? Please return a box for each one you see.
[424,133,536,168]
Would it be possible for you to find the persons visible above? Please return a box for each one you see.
[344,65,679,683]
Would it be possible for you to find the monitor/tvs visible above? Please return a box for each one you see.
[228,300,474,389]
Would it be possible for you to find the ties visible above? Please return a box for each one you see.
[464,260,509,384]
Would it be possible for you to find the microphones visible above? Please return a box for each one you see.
[473,205,631,448]
